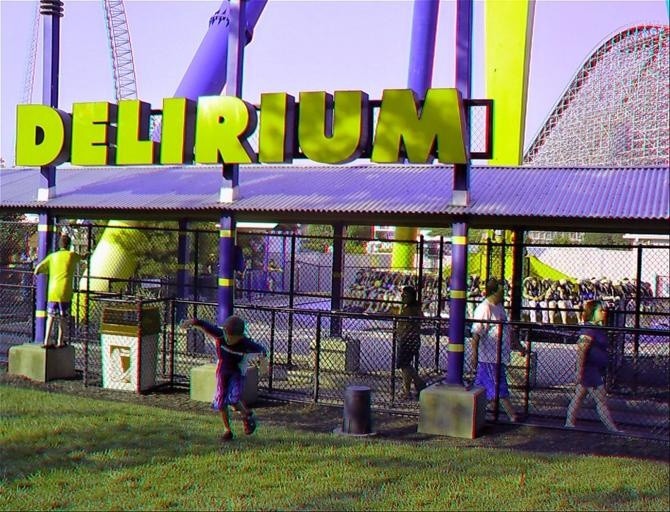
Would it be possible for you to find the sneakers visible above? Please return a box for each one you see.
[242,410,256,436]
[220,431,233,443]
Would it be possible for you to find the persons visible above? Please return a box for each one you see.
[564,300,625,437]
[33,234,91,348]
[471,278,528,423]
[4,247,38,285]
[181,316,267,444]
[204,253,284,298]
[394,286,426,399]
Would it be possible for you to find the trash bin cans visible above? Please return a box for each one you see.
[99,305,160,394]
